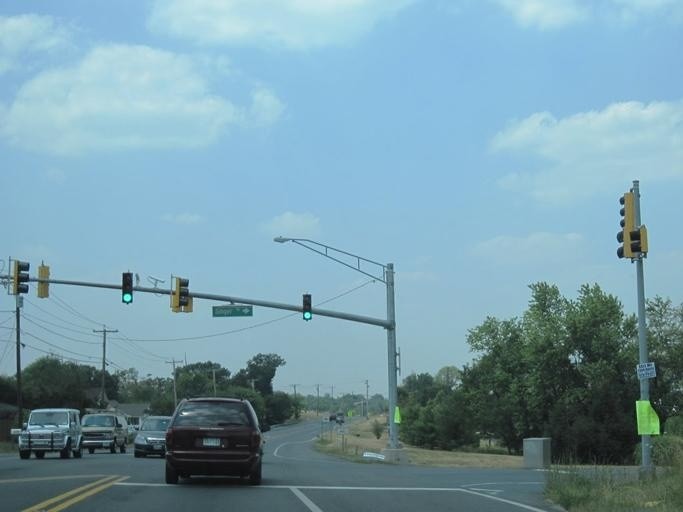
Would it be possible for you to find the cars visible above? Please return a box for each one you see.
[131,416,173,459]
[162,393,264,488]
[322,410,345,425]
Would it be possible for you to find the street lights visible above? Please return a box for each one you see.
[270,232,406,465]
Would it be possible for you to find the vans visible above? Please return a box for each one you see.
[74,411,129,455]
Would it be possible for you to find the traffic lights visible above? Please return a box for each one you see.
[121,273,133,304]
[617,191,633,229]
[174,277,189,314]
[13,258,28,296]
[628,224,648,257]
[613,230,635,260]
[302,294,312,322]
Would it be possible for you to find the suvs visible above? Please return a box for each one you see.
[16,408,83,461]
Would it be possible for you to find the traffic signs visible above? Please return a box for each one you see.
[636,363,657,380]
[211,305,252,318]
